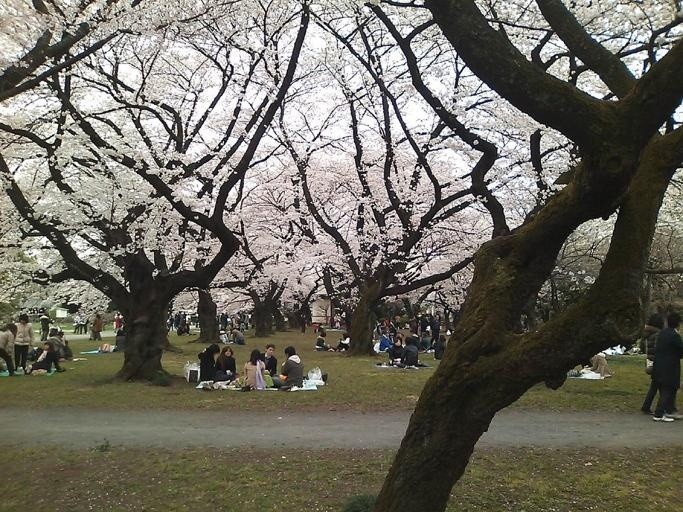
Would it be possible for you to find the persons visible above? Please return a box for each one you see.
[198,343,304,391]
[372,309,451,369]
[652,313,683,422]
[166,309,257,346]
[585,351,615,379]
[299,312,350,353]
[641,314,679,416]
[0,308,123,377]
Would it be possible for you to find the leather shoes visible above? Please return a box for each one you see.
[652,415,674,422]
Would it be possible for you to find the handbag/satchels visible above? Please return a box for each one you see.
[645,358,653,374]
